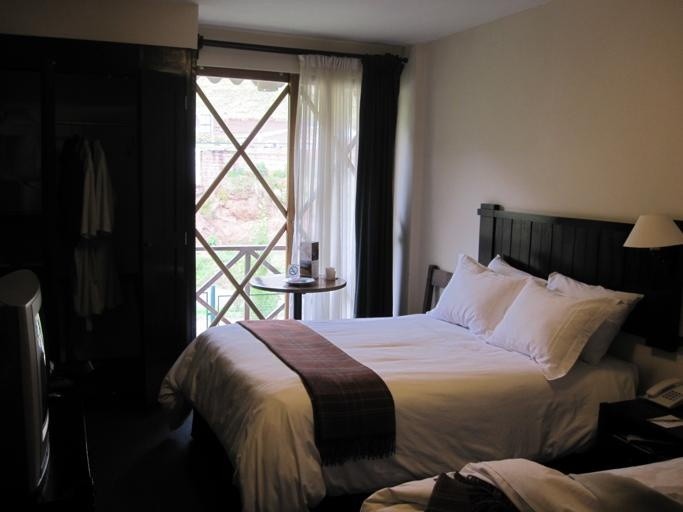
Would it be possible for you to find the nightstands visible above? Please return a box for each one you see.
[595,397,682,467]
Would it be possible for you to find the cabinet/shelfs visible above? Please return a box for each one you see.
[0,32,196,420]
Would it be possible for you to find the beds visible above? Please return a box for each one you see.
[358,455,681,509]
[190,205,682,509]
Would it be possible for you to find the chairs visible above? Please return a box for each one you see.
[422,264,453,315]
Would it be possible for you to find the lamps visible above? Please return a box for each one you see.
[622,213,682,251]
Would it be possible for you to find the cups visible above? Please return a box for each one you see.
[325,267,336,282]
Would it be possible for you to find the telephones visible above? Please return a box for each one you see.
[644,377,683,409]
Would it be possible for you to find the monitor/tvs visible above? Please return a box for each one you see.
[0,269,51,493]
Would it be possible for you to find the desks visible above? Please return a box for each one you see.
[42,365,105,488]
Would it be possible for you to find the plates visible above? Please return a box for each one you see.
[281,277,316,286]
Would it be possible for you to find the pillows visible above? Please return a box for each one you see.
[484,276,623,381]
[427,254,531,341]
[486,254,548,288]
[546,270,644,368]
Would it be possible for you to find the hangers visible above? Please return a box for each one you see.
[83,124,103,157]
